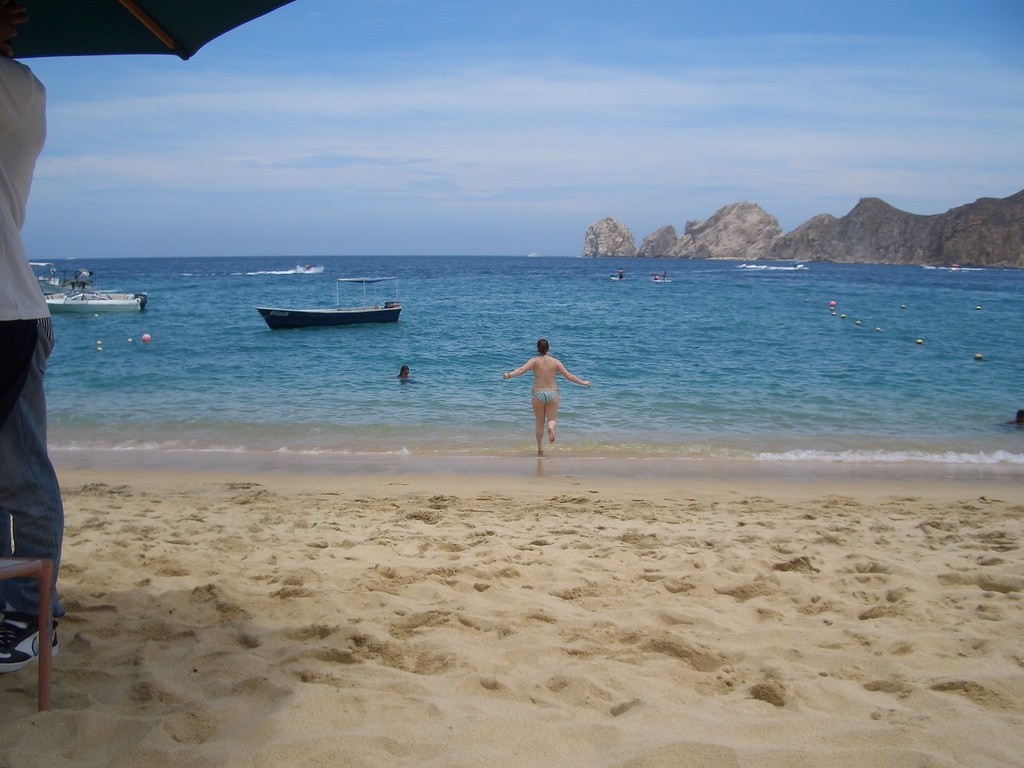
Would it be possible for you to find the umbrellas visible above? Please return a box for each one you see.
[1,0,295,60]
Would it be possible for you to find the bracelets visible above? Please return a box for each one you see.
[508,373,510,378]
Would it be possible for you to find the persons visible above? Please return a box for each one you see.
[662,271,667,279]
[618,268,624,279]
[0,60,64,674]
[654,274,659,280]
[398,366,409,378]
[503,339,591,452]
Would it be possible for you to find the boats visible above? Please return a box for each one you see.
[299,265,325,274]
[254,275,403,330]
[43,269,149,314]
[652,278,671,283]
[795,264,805,269]
[29,261,60,285]
[37,269,98,296]
[951,264,961,271]
[608,272,625,281]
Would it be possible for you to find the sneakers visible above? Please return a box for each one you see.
[0,601,59,673]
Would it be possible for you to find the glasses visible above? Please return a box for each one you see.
[405,372,409,374]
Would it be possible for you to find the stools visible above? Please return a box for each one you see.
[0,557,53,713]
